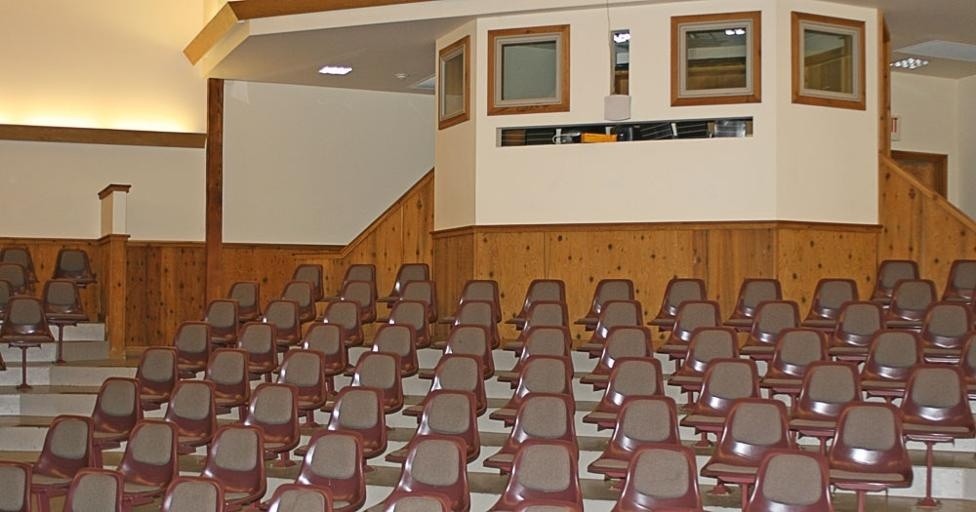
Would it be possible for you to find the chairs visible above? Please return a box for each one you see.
[1,241,973,511]
